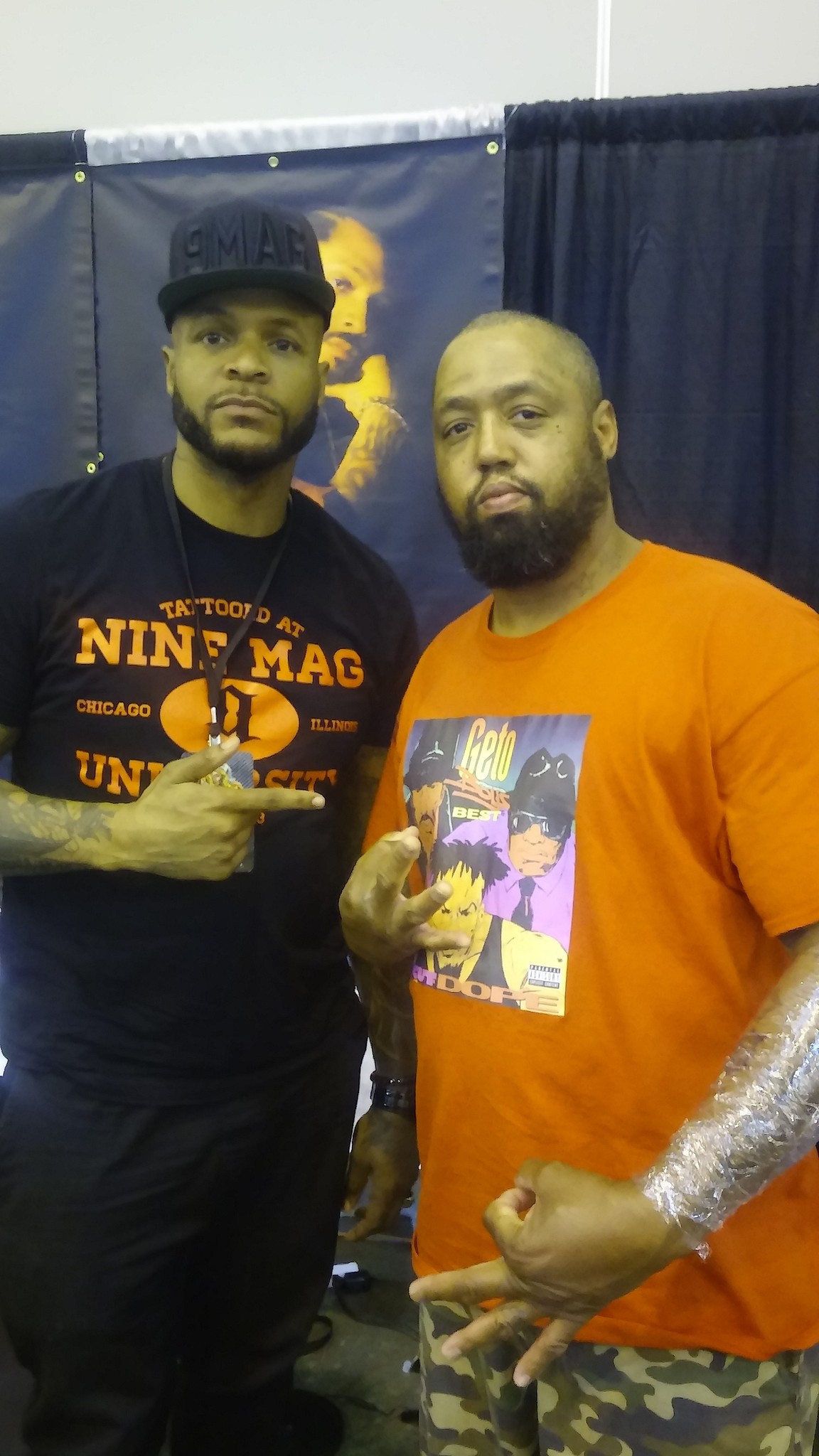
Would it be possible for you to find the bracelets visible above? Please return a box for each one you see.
[370,1070,417,1117]
[355,400,415,437]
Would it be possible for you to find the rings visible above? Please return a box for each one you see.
[405,1191,416,1204]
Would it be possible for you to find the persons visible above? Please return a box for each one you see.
[0,202,417,1456]
[340,311,817,1452]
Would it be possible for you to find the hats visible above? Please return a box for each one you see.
[157,199,335,316]
